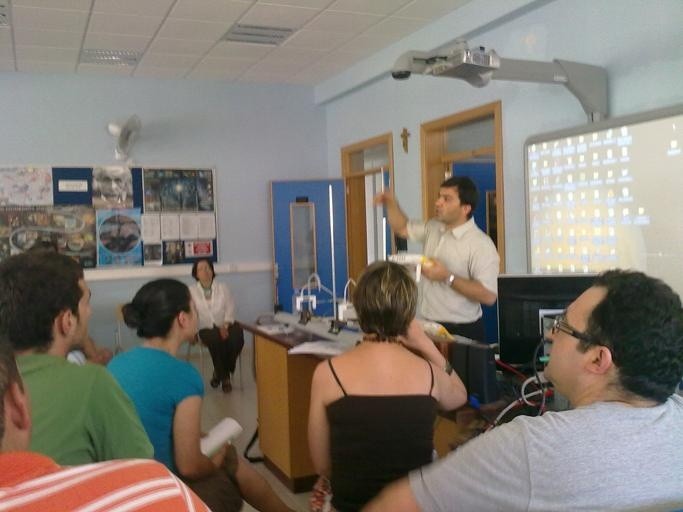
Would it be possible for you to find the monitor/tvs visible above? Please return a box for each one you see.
[497,273,602,365]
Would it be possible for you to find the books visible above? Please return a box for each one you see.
[285,339,344,357]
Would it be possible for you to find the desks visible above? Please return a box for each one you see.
[240,321,449,493]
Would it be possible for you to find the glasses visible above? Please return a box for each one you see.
[551,315,616,362]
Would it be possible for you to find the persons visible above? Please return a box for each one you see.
[0,252,152,466]
[350,270,681,512]
[0,323,214,510]
[104,278,292,511]
[307,262,467,512]
[372,174,500,348]
[186,258,245,394]
[93,165,131,206]
[119,222,138,251]
[101,222,117,247]
[29,242,111,364]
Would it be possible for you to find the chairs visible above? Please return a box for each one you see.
[187,330,248,392]
[114,304,127,351]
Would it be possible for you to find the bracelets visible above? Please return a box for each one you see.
[446,273,455,287]
[442,359,454,377]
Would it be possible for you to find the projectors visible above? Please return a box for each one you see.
[433,49,501,75]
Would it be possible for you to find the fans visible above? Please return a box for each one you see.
[108,113,141,161]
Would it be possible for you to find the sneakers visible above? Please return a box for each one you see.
[211,371,220,387]
[222,376,231,392]
